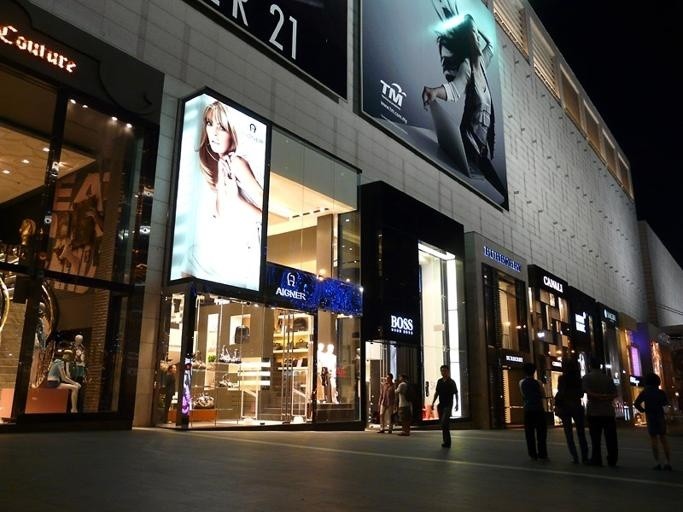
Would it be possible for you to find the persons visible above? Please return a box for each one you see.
[431,365,458,449]
[160,365,176,424]
[325,343,340,404]
[558,357,589,465]
[582,357,618,467]
[519,363,550,462]
[181,103,263,283]
[317,343,325,404]
[47,350,81,413]
[395,375,410,436]
[72,335,85,379]
[421,1,508,207]
[377,374,397,434]
[634,373,675,472]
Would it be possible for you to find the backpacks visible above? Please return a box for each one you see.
[293,318,307,332]
[234,326,249,344]
[403,380,418,401]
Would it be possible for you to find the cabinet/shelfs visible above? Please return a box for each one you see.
[272,311,309,370]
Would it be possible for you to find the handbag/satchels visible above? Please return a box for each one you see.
[284,358,298,367]
[301,357,308,366]
[272,338,308,350]
[192,395,215,409]
[219,344,242,364]
[192,350,207,369]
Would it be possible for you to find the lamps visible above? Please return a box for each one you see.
[315,269,364,296]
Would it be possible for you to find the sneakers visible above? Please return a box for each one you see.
[397,432,411,436]
[652,463,673,472]
[377,429,394,434]
